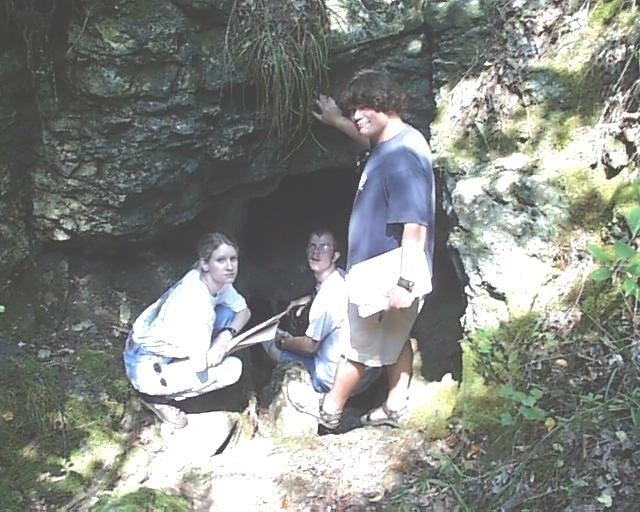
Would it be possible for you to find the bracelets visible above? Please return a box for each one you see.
[397,276,414,293]
[222,327,238,337]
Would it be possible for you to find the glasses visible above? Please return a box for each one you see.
[154,364,166,386]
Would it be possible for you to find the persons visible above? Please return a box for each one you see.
[283,71,439,430]
[259,226,385,399]
[121,227,251,427]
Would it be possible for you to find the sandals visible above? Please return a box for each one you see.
[288,381,344,429]
[360,402,407,427]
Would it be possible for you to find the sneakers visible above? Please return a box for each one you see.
[141,398,187,428]
